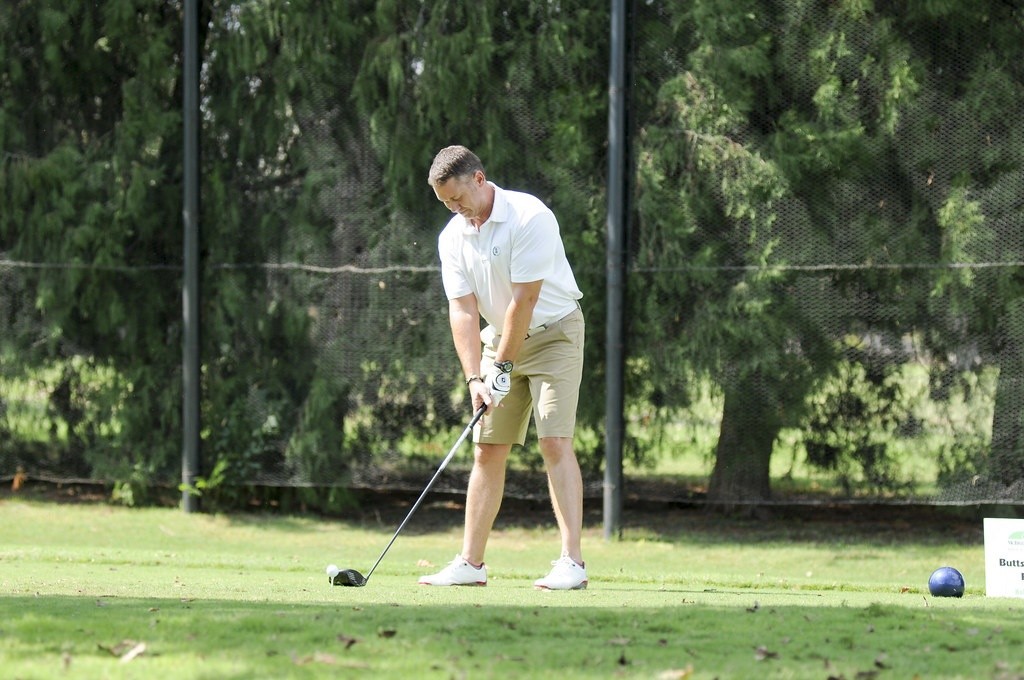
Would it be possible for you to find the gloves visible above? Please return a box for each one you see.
[483,366,510,407]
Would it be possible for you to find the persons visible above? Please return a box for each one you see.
[418,146,590,590]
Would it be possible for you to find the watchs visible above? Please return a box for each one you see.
[495,360,514,373]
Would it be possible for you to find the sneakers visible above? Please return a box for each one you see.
[418,553,487,586]
[535,551,588,590]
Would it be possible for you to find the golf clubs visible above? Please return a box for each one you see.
[329,401,488,587]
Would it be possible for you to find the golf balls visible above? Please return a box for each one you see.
[326,564,339,578]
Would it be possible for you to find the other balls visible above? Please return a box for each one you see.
[928,566,965,599]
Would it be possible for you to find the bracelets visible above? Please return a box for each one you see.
[466,375,485,387]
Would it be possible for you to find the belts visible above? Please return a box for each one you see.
[524,301,578,340]
